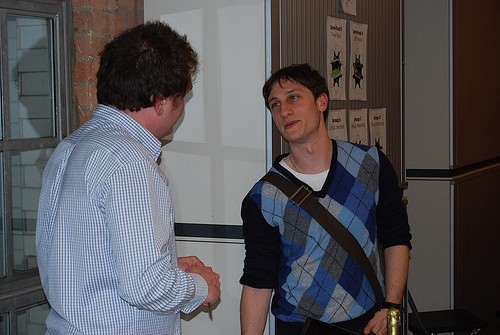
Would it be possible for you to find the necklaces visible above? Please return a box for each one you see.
[288,153,301,174]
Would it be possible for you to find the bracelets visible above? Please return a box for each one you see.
[380,300,404,314]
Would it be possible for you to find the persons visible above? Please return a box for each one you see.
[34,20,221,335]
[239,62,412,335]
